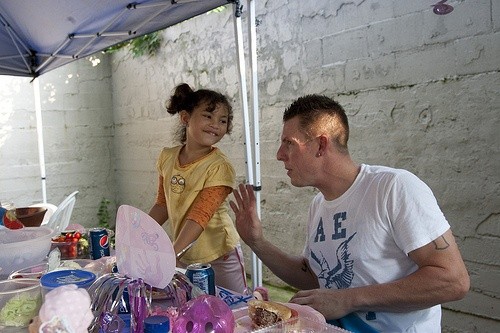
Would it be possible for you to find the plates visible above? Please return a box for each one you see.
[7,259,94,286]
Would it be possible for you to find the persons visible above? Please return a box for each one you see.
[230,93,469,333]
[147,82,248,295]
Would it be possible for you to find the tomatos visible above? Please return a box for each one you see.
[54,232,81,260]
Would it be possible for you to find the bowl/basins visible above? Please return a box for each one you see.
[51,227,115,260]
[0,278,42,333]
[14,207,48,227]
[0,226,53,276]
[39,269,97,304]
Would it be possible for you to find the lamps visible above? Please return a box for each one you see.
[431,0,454,15]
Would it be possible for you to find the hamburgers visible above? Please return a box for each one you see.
[246,299,300,333]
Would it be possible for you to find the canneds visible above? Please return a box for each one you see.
[89,228,110,259]
[184,262,216,301]
